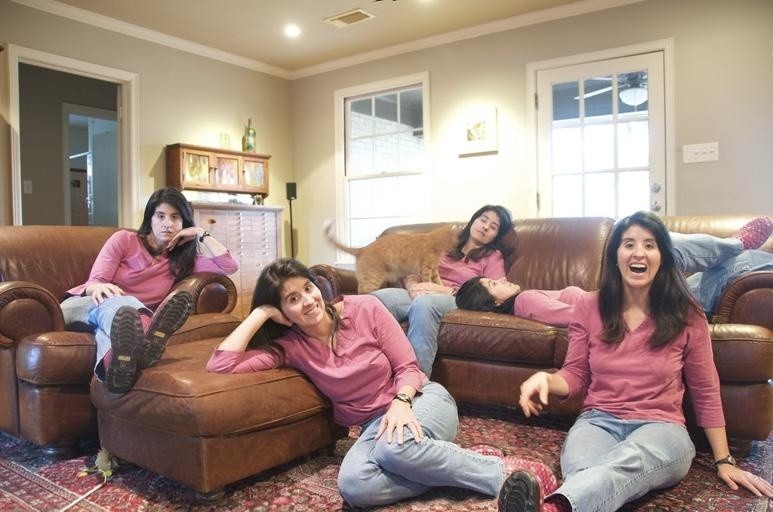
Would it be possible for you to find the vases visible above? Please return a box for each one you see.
[242,118,257,152]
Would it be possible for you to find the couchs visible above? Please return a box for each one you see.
[308,213,773,459]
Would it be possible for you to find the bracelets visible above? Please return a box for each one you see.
[450,287,458,295]
[199,231,210,241]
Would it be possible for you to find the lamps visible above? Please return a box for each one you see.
[286,182,298,256]
[617,84,650,107]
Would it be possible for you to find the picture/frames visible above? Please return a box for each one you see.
[457,106,498,156]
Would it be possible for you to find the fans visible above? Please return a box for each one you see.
[574,71,648,101]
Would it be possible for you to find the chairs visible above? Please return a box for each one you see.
[0,225,244,462]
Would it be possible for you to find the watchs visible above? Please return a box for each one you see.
[715,453,737,467]
[393,393,412,405]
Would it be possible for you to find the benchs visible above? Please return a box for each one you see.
[91,337,347,502]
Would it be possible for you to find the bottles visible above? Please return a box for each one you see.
[243,118,255,152]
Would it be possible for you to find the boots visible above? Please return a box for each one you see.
[733,215,773,250]
[103,305,145,394]
[138,289,193,370]
[462,443,571,512]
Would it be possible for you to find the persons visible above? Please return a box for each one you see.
[367,205,519,378]
[497,214,772,512]
[59,188,237,394]
[206,258,556,508]
[453,217,772,328]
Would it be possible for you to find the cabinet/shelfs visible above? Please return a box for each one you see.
[188,201,285,318]
[166,144,273,198]
